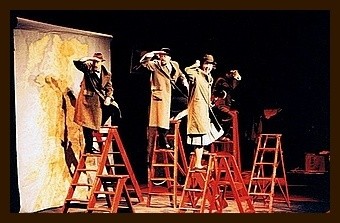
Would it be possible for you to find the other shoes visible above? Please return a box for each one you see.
[194,164,206,169]
[220,136,230,142]
[159,141,174,150]
[84,147,101,154]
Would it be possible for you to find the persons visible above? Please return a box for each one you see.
[184,53,224,169]
[140,48,189,149]
[73,52,120,154]
[210,70,241,152]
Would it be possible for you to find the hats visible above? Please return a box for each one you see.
[230,70,241,80]
[158,48,170,54]
[93,52,106,61]
[200,54,217,64]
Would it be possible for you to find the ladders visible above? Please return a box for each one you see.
[63,126,144,213]
[85,174,133,213]
[248,134,290,213]
[144,111,255,212]
